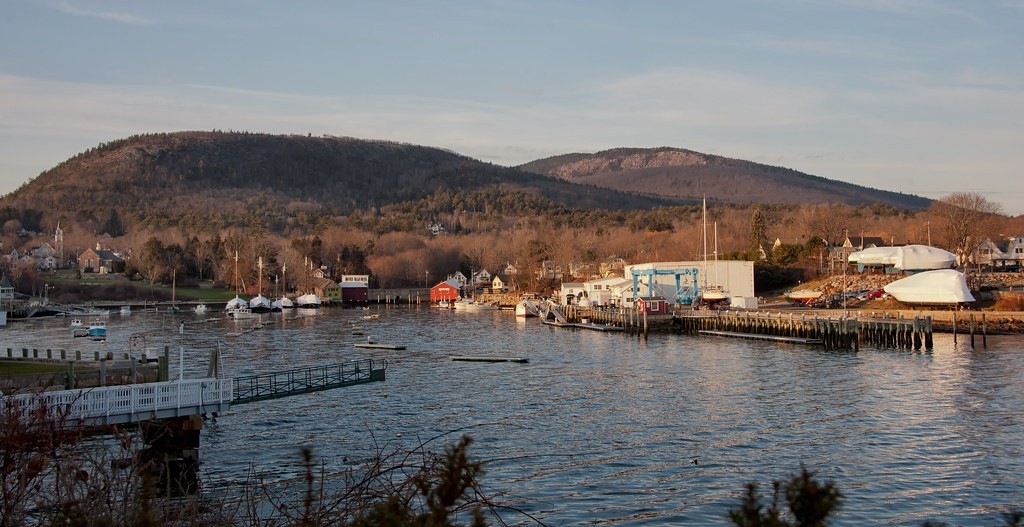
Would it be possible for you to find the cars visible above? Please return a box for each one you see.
[846,290,869,299]
[866,289,885,300]
[856,291,872,299]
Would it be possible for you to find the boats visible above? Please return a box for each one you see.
[783,289,823,303]
[88,320,107,336]
[229,309,255,320]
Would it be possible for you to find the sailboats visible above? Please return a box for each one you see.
[224,250,249,312]
[280,262,294,308]
[271,275,283,312]
[696,192,731,303]
[249,256,271,312]
[297,256,322,308]
